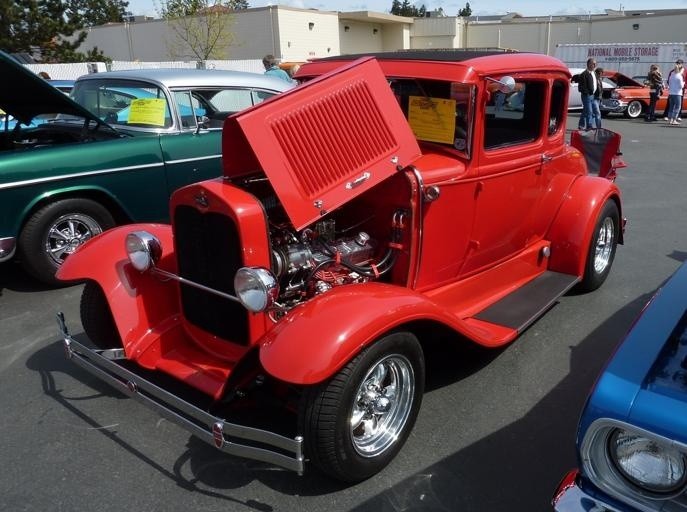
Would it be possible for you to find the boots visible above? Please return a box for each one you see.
[650,115,656,120]
[644,115,651,122]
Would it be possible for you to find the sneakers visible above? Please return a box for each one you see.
[663,117,681,124]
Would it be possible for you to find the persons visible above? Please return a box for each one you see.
[289,65,301,78]
[663,60,687,121]
[578,59,599,130]
[258,55,298,101]
[592,68,603,128]
[644,65,665,122]
[649,66,664,95]
[668,64,685,125]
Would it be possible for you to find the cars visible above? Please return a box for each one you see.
[568,67,687,119]
[549,254,687,508]
[55,52,627,476]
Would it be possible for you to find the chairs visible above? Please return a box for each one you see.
[485,118,527,141]
